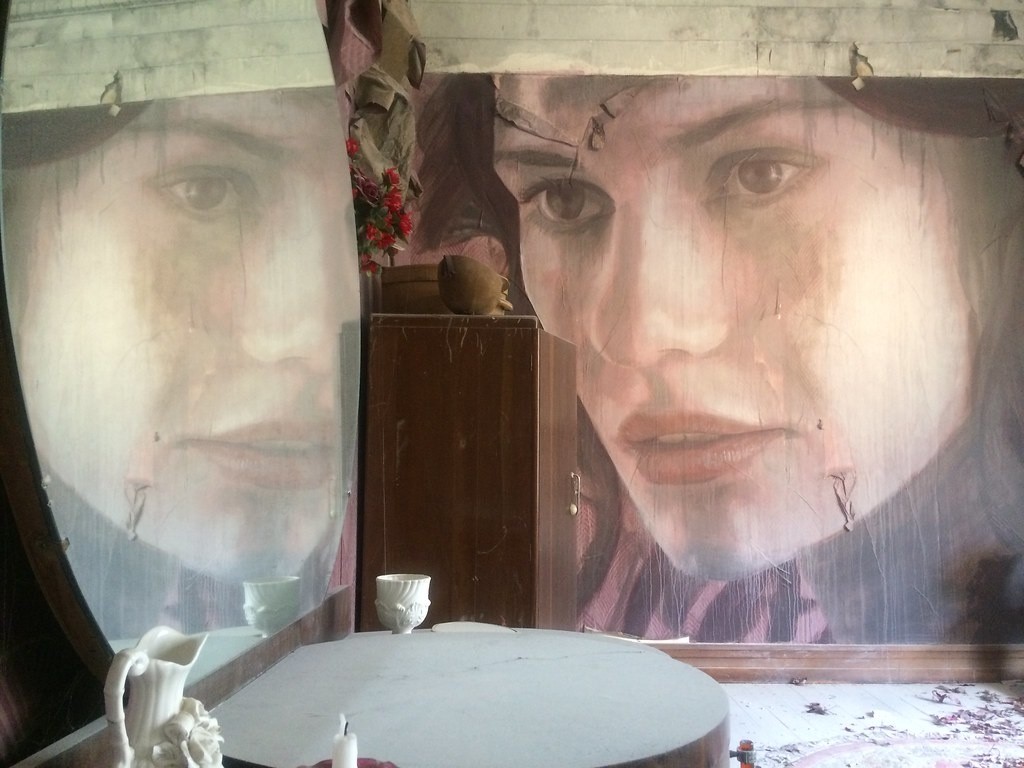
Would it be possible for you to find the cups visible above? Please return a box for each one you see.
[375,573,432,634]
[242,575,301,641]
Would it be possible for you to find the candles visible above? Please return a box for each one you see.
[332,714,359,768]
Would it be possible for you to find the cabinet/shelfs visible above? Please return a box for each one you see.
[359,312,538,629]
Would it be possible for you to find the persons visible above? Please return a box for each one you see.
[411,75,1024,647]
[1,87,361,766]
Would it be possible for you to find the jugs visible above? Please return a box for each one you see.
[105,627,226,768]
